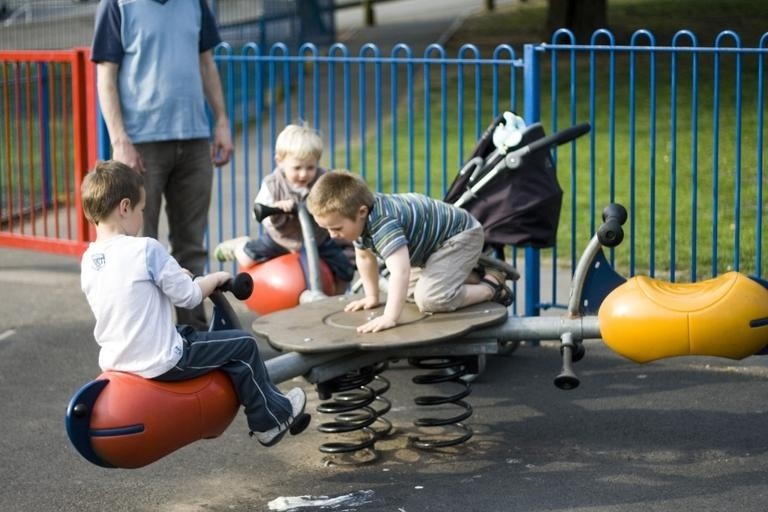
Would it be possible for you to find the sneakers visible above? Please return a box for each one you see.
[215,237,251,261]
[250,388,306,446]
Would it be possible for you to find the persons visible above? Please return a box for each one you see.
[78,158,308,449]
[305,165,522,336]
[88,0,235,331]
[214,116,357,281]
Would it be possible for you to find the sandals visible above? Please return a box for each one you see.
[473,257,520,306]
